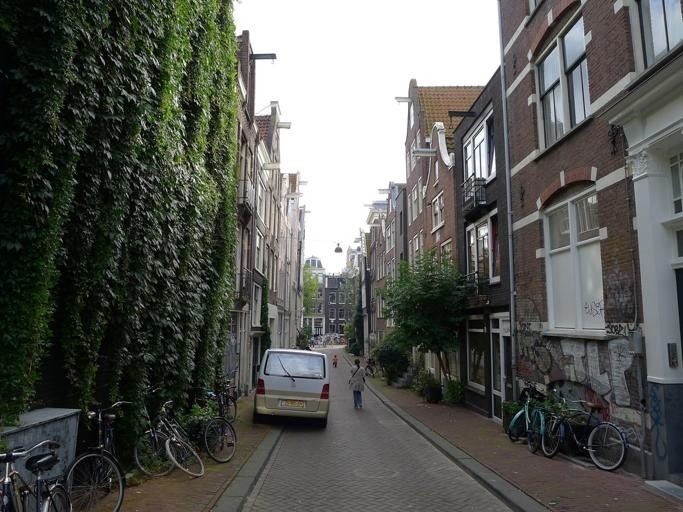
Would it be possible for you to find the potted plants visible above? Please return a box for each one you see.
[500,401,531,439]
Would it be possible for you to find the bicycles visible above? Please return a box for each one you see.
[199,392,236,463]
[540,388,629,471]
[364,358,376,377]
[126,387,186,478]
[59,400,132,511]
[151,399,204,477]
[506,377,545,454]
[216,369,238,424]
[0,440,72,512]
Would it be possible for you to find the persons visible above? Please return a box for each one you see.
[347,358,365,409]
[331,355,337,368]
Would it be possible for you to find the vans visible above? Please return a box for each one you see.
[252,348,329,428]
